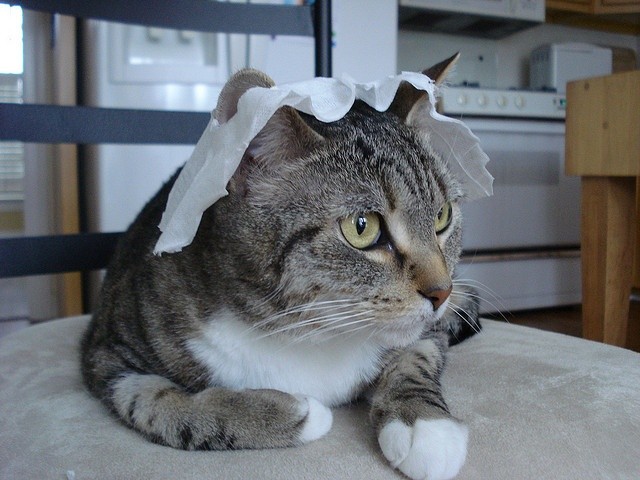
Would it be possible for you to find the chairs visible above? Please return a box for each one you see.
[0,2,639,480]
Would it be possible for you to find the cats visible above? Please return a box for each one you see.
[79,58,512,475]
